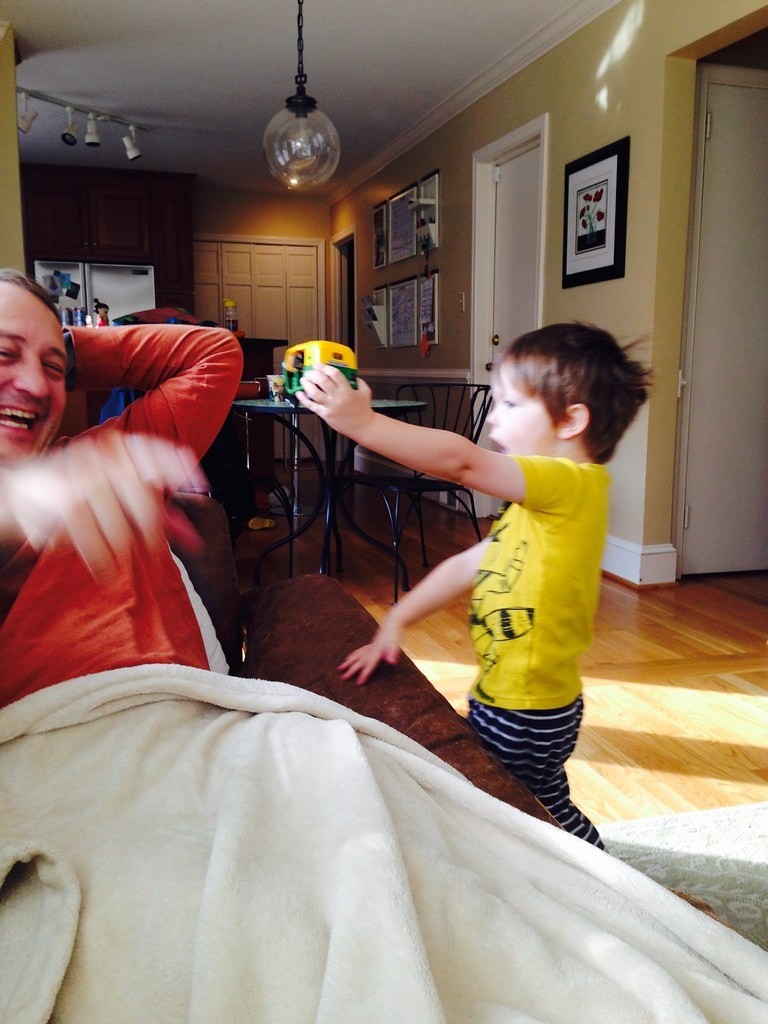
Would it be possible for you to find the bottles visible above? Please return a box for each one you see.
[223,298,238,332]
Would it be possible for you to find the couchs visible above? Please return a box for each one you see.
[171,491,565,829]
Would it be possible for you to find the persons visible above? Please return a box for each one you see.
[1,266,241,741]
[294,319,652,855]
[95,300,282,542]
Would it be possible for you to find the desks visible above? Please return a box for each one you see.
[220,338,286,490]
[233,400,431,592]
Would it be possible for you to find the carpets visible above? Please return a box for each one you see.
[594,801,768,951]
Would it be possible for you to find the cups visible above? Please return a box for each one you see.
[267,375,286,402]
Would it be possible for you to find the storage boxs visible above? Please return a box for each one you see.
[34,260,86,309]
[85,262,156,325]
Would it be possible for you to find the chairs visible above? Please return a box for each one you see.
[343,381,492,607]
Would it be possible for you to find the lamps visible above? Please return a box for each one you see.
[123,125,142,161]
[60,108,81,146]
[18,92,38,134]
[262,0,341,193]
[85,113,111,147]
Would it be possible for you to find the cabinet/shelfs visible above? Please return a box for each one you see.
[20,162,198,315]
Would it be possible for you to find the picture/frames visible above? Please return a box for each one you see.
[418,274,441,344]
[372,204,386,270]
[387,187,418,264]
[561,134,631,289]
[390,279,418,348]
